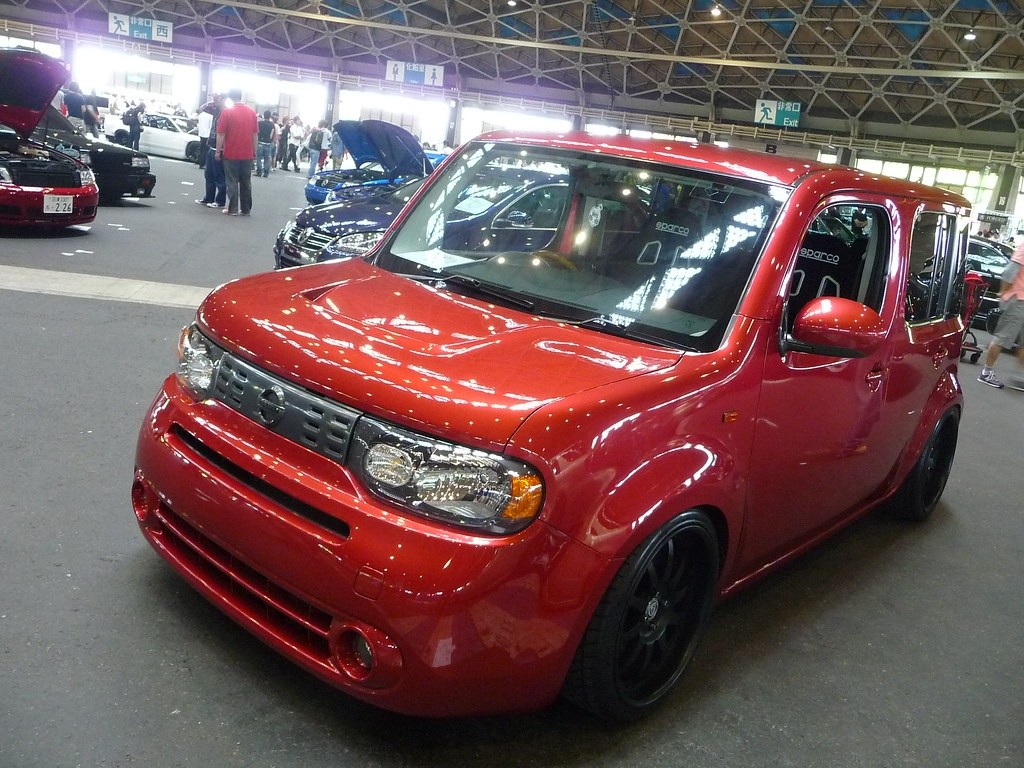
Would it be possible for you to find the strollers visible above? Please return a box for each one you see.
[960,269,996,365]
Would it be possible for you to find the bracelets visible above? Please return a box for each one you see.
[216,152,221,153]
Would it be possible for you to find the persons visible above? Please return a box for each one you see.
[214,88,259,217]
[51,88,65,115]
[82,89,102,139]
[976,228,1015,249]
[128,103,147,151]
[63,81,87,136]
[977,237,1024,392]
[195,92,227,208]
[849,208,868,235]
[650,175,671,216]
[252,110,348,179]
[412,135,460,156]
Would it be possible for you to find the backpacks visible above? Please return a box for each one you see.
[123,108,134,125]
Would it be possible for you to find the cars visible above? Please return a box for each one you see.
[967,235,1017,334]
[0,48,100,227]
[303,119,502,208]
[125,132,972,726]
[1,104,157,205]
[103,112,206,165]
[273,118,652,273]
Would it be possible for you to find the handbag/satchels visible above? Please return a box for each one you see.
[87,108,99,124]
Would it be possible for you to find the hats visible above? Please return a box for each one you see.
[211,91,227,99]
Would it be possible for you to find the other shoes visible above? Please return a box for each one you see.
[195,199,208,205]
[253,173,261,177]
[206,202,225,209]
[283,168,291,171]
[295,168,300,173]
[238,210,249,216]
[263,173,268,177]
[222,209,238,216]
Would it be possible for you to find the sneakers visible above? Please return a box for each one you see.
[976,370,1004,388]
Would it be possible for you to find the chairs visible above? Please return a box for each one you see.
[603,208,701,278]
[788,233,849,339]
